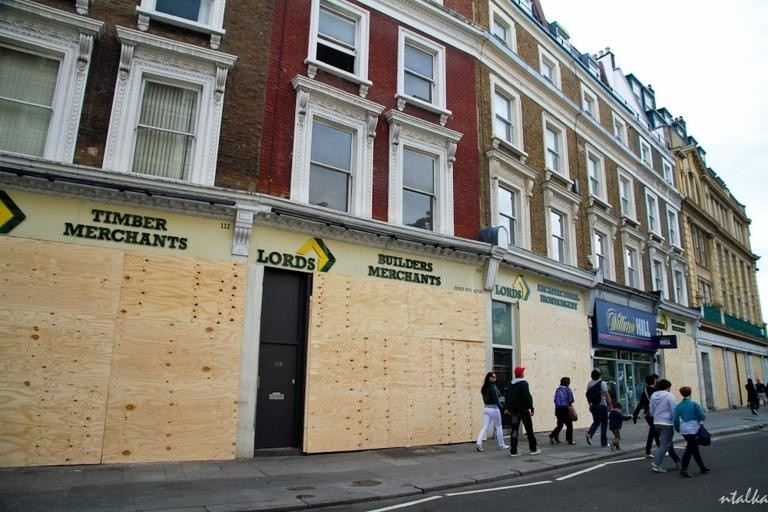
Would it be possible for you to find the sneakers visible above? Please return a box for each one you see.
[476,445,484,452]
[567,440,576,445]
[645,451,712,479]
[753,408,759,416]
[585,434,593,446]
[528,448,541,455]
[548,434,555,445]
[606,442,621,452]
[497,445,510,450]
[510,452,523,457]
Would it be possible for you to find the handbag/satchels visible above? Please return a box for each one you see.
[584,380,603,405]
[568,404,577,422]
[694,424,712,447]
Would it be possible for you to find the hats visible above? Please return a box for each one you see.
[514,367,525,376]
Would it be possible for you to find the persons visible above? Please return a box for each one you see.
[505,368,542,457]
[476,372,511,451]
[548,376,578,445]
[653,373,660,381]
[765,383,768,405]
[648,379,682,473]
[755,379,767,408]
[608,402,634,451]
[586,368,614,447]
[673,386,711,477]
[555,430,567,442]
[744,378,760,416]
[633,375,669,457]
